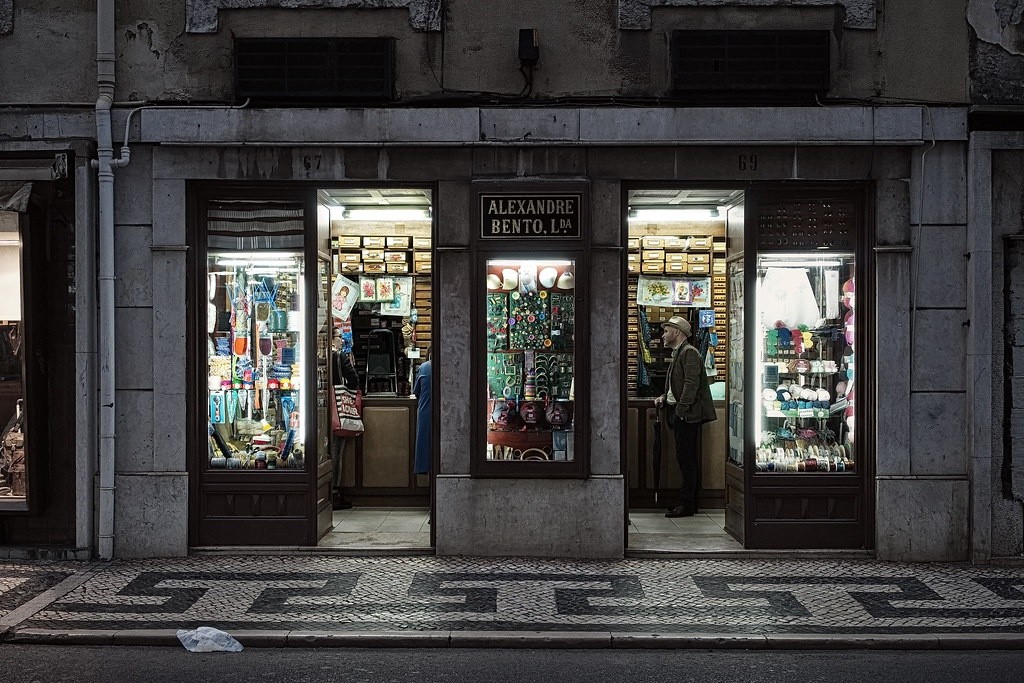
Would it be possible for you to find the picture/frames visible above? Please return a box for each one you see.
[380,276,413,317]
[376,278,397,303]
[332,273,360,322]
[357,275,376,303]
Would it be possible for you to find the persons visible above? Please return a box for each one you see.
[654,315,716,517]
[331,326,360,510]
[414,344,432,474]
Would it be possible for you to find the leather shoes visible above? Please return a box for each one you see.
[665,505,699,518]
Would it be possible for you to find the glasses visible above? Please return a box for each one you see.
[332,334,342,338]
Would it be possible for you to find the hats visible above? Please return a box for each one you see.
[661,316,693,337]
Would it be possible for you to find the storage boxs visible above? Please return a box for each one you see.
[629,237,726,389]
[331,236,431,371]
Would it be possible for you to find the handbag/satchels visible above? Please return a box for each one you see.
[332,350,365,437]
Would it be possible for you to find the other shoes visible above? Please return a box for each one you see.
[332,499,352,510]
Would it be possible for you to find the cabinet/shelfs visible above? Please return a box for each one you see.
[628,238,726,389]
[763,329,840,447]
[330,219,432,394]
